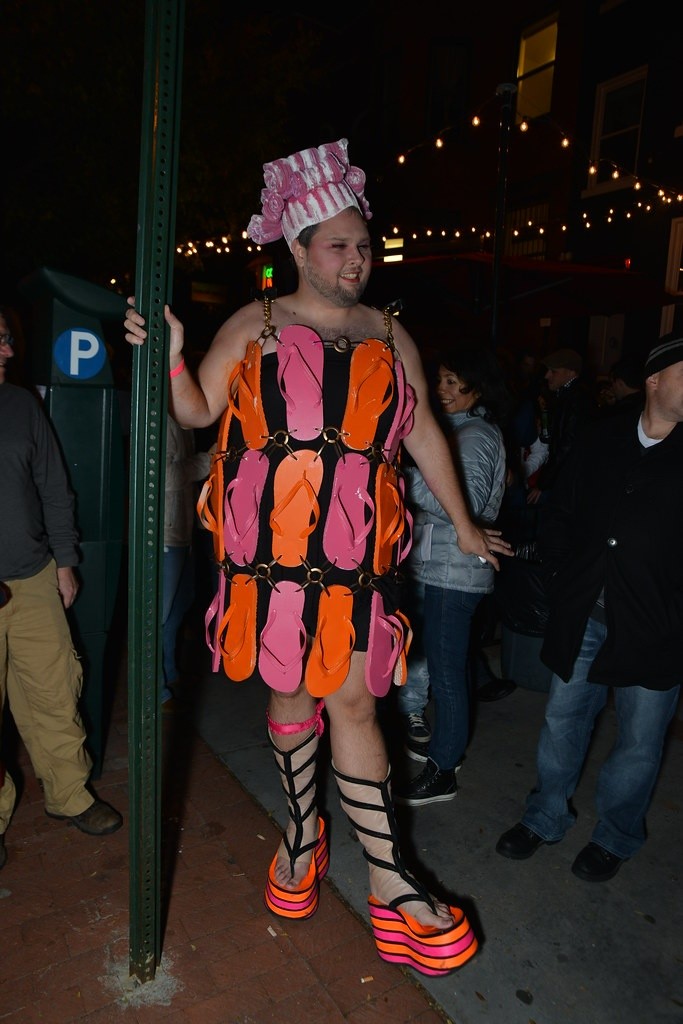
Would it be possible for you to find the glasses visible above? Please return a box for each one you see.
[0,334,15,346]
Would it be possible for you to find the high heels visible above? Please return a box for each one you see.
[331,759,477,978]
[264,725,330,920]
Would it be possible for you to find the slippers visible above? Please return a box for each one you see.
[196,321,417,699]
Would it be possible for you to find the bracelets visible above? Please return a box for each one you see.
[169,358,184,378]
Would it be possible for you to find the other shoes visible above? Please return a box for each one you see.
[472,678,516,701]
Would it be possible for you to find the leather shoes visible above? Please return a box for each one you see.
[495,822,559,862]
[572,841,622,882]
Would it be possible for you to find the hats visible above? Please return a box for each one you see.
[540,348,583,371]
[642,330,682,382]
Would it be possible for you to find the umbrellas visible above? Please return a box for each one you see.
[281,252,683,347]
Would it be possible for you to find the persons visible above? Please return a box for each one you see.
[398,354,508,804]
[1,313,121,868]
[125,138,517,978]
[162,411,216,732]
[496,328,683,882]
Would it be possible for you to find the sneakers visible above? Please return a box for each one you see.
[0,832,8,868]
[45,799,123,835]
[401,740,462,771]
[390,756,459,807]
[400,709,432,743]
